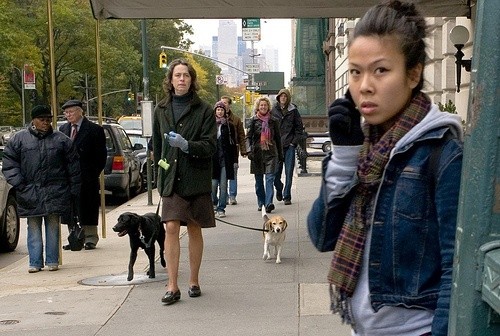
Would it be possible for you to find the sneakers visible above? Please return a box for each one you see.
[230,198,237,205]
[28,266,41,273]
[217,212,226,218]
[49,264,59,271]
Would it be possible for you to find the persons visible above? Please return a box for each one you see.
[211,101,239,217]
[221,96,246,205]
[246,97,283,213]
[270,89,302,205]
[2,105,83,272]
[152,59,217,303]
[59,100,107,250]
[307,0,464,336]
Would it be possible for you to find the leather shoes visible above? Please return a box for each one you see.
[161,289,180,303]
[188,285,201,297]
[85,242,96,250]
[266,203,275,213]
[276,183,283,201]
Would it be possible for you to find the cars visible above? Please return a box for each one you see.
[0,125,16,146]
[0,166,20,252]
[308,131,332,152]
[117,114,150,147]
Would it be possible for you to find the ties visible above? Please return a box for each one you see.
[71,124,78,140]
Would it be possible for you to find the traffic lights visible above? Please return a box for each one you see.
[159,50,167,69]
[128,92,132,101]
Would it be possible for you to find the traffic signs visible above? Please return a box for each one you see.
[246,85,261,91]
[245,64,260,73]
[23,63,36,88]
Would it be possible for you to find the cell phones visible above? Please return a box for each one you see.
[345,89,361,116]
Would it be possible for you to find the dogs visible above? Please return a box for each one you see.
[262,205,288,264]
[112,212,166,281]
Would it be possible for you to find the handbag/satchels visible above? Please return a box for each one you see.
[68,197,85,251]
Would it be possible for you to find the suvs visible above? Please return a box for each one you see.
[87,116,144,201]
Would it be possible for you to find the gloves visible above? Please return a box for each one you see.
[328,98,365,145]
[164,131,189,154]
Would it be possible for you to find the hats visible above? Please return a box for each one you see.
[284,199,291,205]
[61,100,82,108]
[31,106,54,119]
[214,101,234,124]
